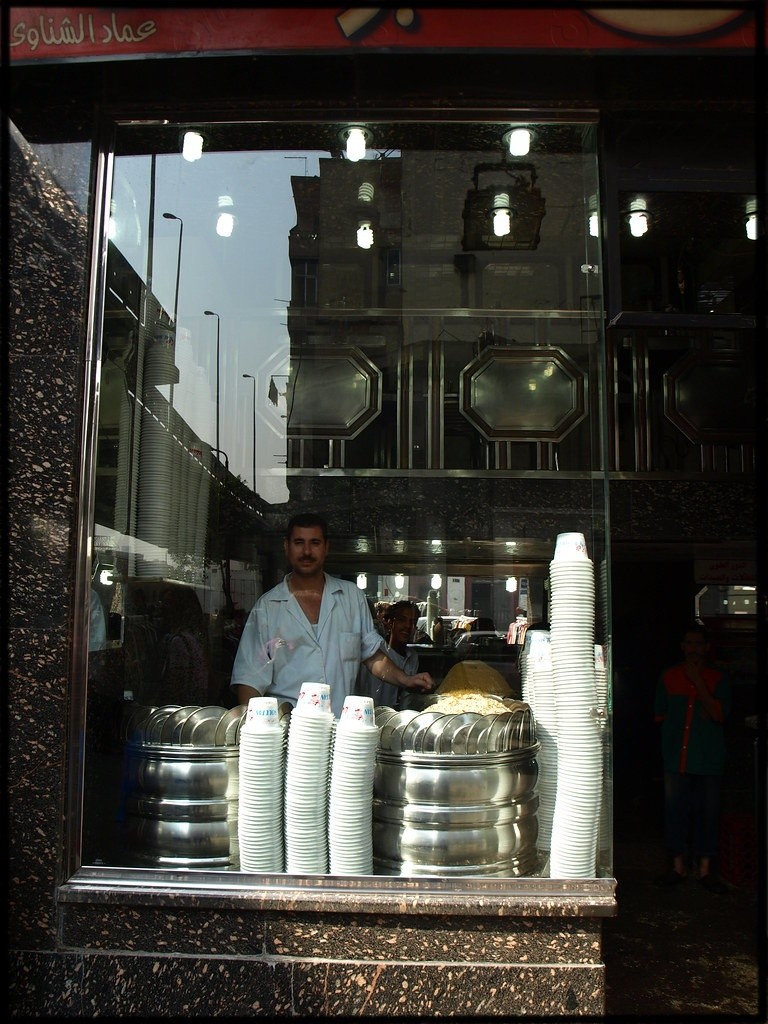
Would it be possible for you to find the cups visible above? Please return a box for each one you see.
[114,276,214,582]
[520,532,606,878]
[240,682,378,879]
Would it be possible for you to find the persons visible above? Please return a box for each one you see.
[88,553,106,653]
[372,602,392,643]
[230,514,436,719]
[355,601,421,710]
[139,585,209,707]
[653,620,732,894]
